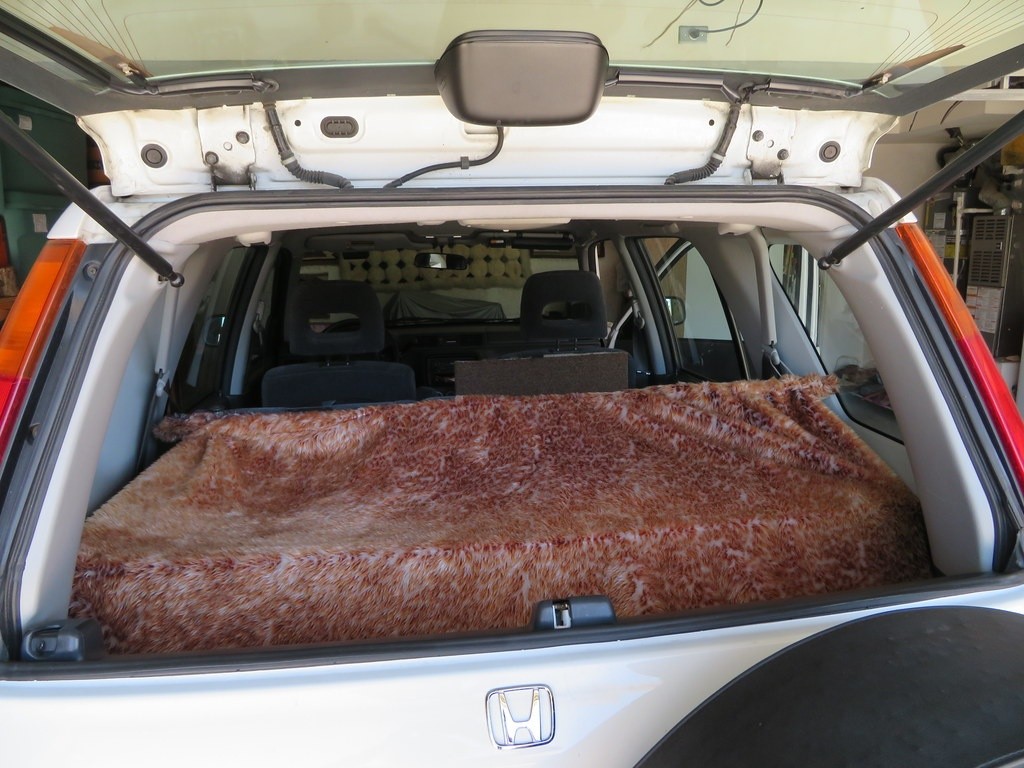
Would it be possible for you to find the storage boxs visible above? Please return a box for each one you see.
[0,87,98,288]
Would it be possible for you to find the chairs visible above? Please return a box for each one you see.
[521,270,633,395]
[260,279,414,405]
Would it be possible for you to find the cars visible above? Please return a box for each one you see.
[0,0,1023,767]
